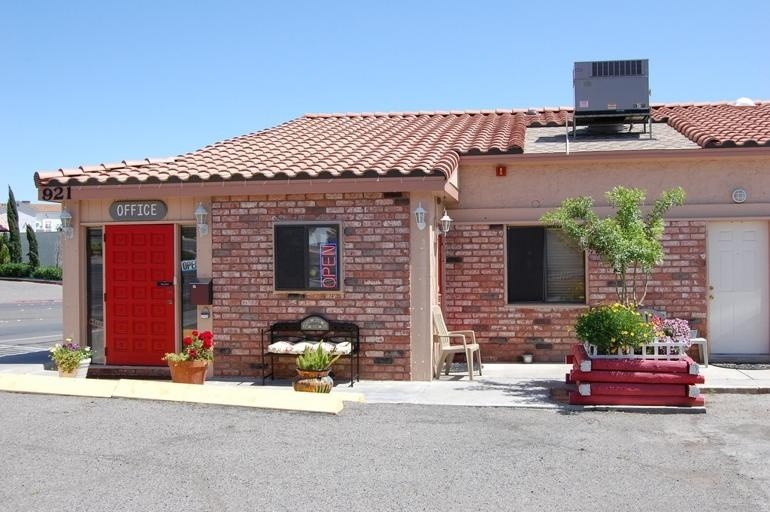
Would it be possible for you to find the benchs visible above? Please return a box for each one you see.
[258,315,360,388]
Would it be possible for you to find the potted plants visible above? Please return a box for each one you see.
[295,340,343,392]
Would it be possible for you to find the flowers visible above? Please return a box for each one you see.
[50,337,94,366]
[163,330,214,360]
[566,300,693,359]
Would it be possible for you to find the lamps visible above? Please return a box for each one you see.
[413,201,428,232]
[439,209,455,239]
[59,205,74,243]
[191,202,210,237]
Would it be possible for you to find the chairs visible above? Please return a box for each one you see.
[431,303,484,382]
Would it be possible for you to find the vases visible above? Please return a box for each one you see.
[58,358,93,378]
[164,359,210,385]
